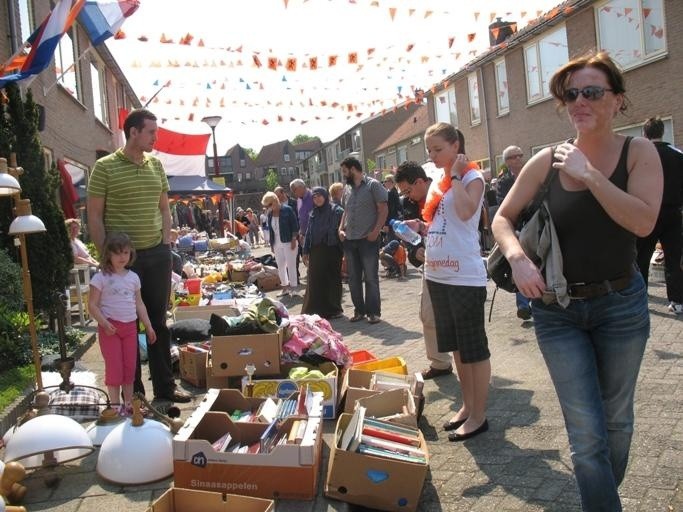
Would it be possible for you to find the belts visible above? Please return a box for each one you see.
[567,264,636,300]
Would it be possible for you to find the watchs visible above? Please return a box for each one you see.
[451,175,462,181]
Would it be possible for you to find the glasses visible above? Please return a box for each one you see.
[505,154,523,160]
[563,85,613,102]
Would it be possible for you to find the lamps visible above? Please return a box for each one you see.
[0,158,174,484]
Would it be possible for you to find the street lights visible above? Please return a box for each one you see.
[203,115,224,238]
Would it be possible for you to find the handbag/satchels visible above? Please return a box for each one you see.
[486,138,575,294]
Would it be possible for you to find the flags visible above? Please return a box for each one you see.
[76,0,140,47]
[0,0,86,88]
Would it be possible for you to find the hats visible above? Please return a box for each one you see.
[385,240,399,251]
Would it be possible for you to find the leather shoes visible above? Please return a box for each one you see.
[422,365,453,380]
[517,306,532,320]
[277,288,297,297]
[443,416,489,441]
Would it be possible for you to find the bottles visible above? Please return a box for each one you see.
[387,219,422,246]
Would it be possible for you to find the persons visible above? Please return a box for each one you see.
[484,145,531,319]
[637,115,683,315]
[206,207,261,249]
[88,231,157,417]
[338,157,407,323]
[259,187,305,297]
[394,160,452,380]
[290,178,348,319]
[422,124,490,440]
[87,108,192,402]
[65,218,100,284]
[491,49,664,512]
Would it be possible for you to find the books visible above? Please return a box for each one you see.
[210,398,427,464]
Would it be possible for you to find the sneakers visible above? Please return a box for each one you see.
[669,302,683,314]
[111,402,147,418]
[170,388,191,402]
[351,312,379,323]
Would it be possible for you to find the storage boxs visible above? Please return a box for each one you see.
[146,237,429,512]
